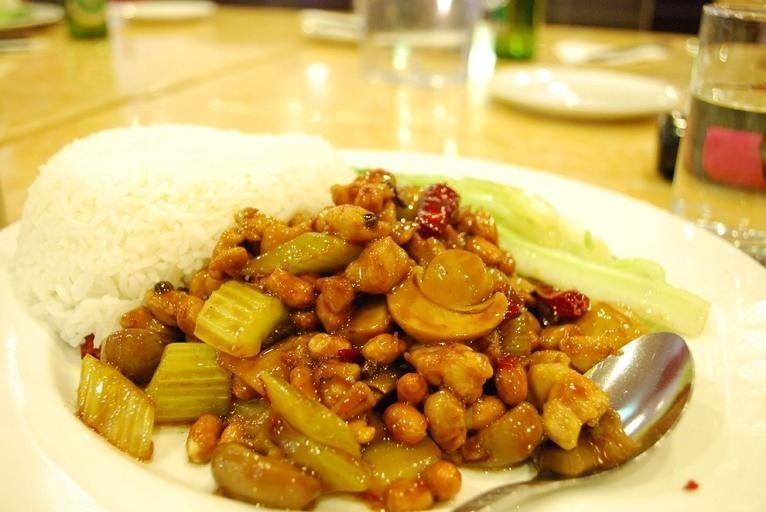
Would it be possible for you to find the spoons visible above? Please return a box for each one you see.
[443,330,694,512]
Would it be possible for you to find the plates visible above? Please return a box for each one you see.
[0,147,766,512]
[489,67,677,124]
[0,0,219,32]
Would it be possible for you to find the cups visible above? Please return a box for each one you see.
[352,0,477,90]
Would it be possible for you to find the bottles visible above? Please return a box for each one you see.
[683,0,766,191]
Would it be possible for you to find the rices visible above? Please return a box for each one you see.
[11,124,358,351]
